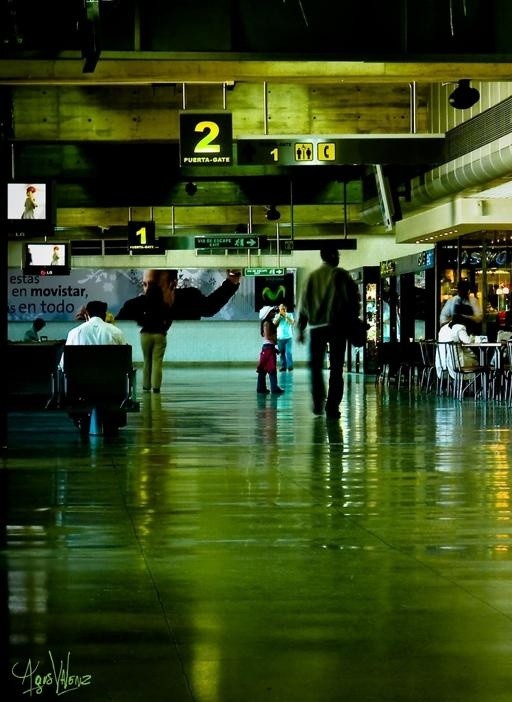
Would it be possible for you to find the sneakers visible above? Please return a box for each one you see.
[326,410,341,418]
[313,405,324,414]
[271,387,284,393]
[257,388,269,393]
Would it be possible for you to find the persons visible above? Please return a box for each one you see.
[297,243,360,418]
[434,313,483,392]
[50,246,61,265]
[255,304,285,395]
[21,186,40,220]
[273,303,296,372]
[60,300,129,445]
[112,269,242,321]
[136,281,173,394]
[439,277,481,325]
[20,317,47,342]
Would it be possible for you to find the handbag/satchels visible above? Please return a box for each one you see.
[344,313,370,348]
[459,349,480,367]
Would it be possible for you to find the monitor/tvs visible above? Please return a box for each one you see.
[1,180,56,241]
[373,163,402,232]
[22,240,71,276]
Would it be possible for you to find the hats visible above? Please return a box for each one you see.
[259,306,274,320]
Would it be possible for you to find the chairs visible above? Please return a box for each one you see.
[374,330,512,407]
[8,343,137,435]
